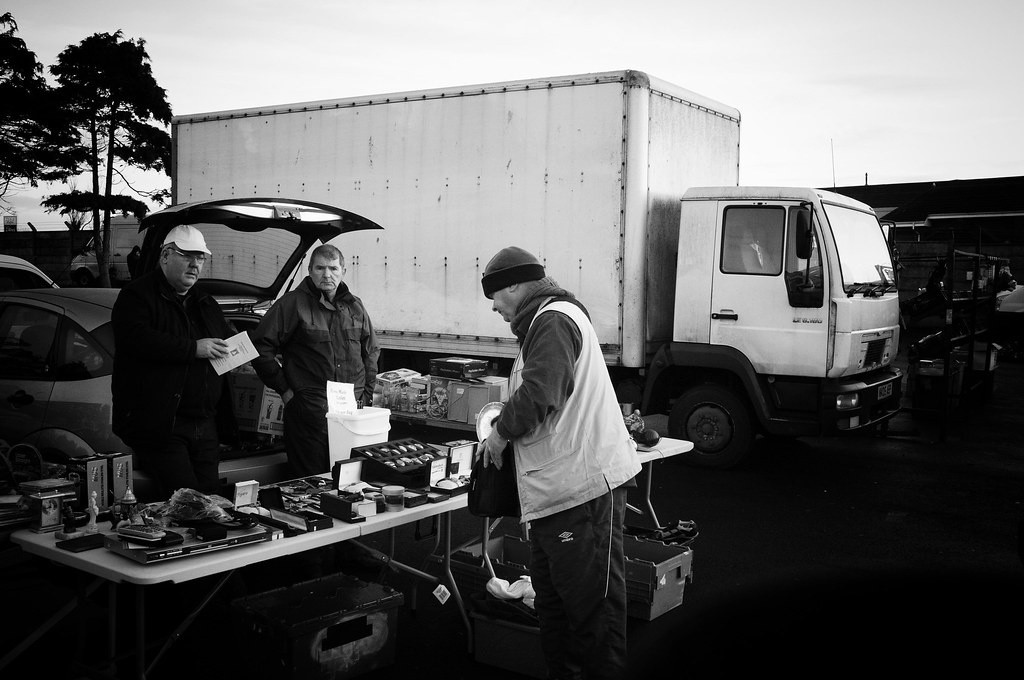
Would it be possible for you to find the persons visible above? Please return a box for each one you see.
[476,245,643,680]
[251,244,381,477]
[111,225,241,501]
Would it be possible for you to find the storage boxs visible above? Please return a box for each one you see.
[232,441,699,679]
[431,357,488,379]
[908,342,1003,409]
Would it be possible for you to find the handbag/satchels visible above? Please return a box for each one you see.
[467,441,522,519]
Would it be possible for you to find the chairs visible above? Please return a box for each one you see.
[725,225,763,273]
[17,325,59,371]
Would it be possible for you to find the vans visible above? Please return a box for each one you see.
[65,214,148,286]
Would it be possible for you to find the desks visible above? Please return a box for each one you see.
[373,406,476,433]
[9,437,695,610]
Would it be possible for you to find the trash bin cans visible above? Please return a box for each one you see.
[908,330,1002,412]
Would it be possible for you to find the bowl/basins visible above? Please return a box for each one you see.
[63,511,90,528]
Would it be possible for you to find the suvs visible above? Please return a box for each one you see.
[0,197,386,494]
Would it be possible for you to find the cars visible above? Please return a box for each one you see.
[0,254,91,347]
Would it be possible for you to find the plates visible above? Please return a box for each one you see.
[476,402,505,442]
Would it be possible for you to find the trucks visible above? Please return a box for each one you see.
[174,70,906,474]
[892,239,1010,350]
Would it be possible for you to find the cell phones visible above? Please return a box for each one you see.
[57,532,105,553]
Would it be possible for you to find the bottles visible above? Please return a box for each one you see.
[382,485,404,513]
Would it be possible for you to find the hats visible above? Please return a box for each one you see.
[482,247,545,299]
[162,224,211,254]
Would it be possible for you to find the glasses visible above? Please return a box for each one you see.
[167,247,207,264]
